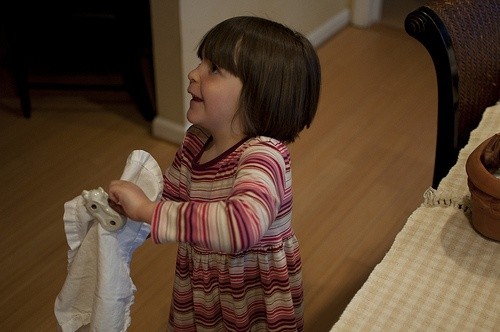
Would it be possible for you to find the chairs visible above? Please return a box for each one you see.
[404,0,500,189]
[0,0,157,123]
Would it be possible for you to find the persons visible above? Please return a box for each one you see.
[109,16,322,332]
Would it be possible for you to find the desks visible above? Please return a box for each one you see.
[329,102,500,332]
